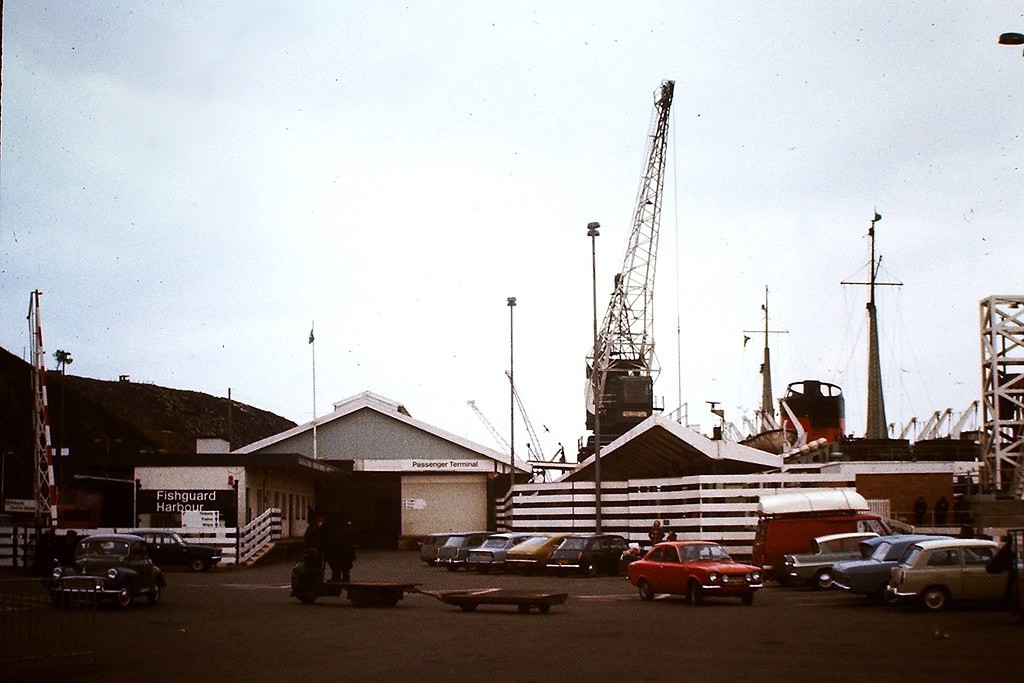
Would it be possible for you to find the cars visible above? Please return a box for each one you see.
[108,527,223,573]
[782,530,882,592]
[830,534,964,606]
[418,531,702,579]
[857,532,993,567]
[626,540,764,607]
[887,538,1024,611]
[43,532,168,610]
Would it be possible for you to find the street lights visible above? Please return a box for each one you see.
[506,296,518,486]
[586,221,604,537]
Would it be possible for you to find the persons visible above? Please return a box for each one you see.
[647,521,676,547]
[933,497,948,527]
[913,496,928,527]
[320,514,355,583]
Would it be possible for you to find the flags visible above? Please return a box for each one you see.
[309,329,315,345]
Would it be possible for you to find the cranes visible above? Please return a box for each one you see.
[884,399,981,445]
[582,77,679,462]
[721,408,784,444]
[465,370,555,484]
